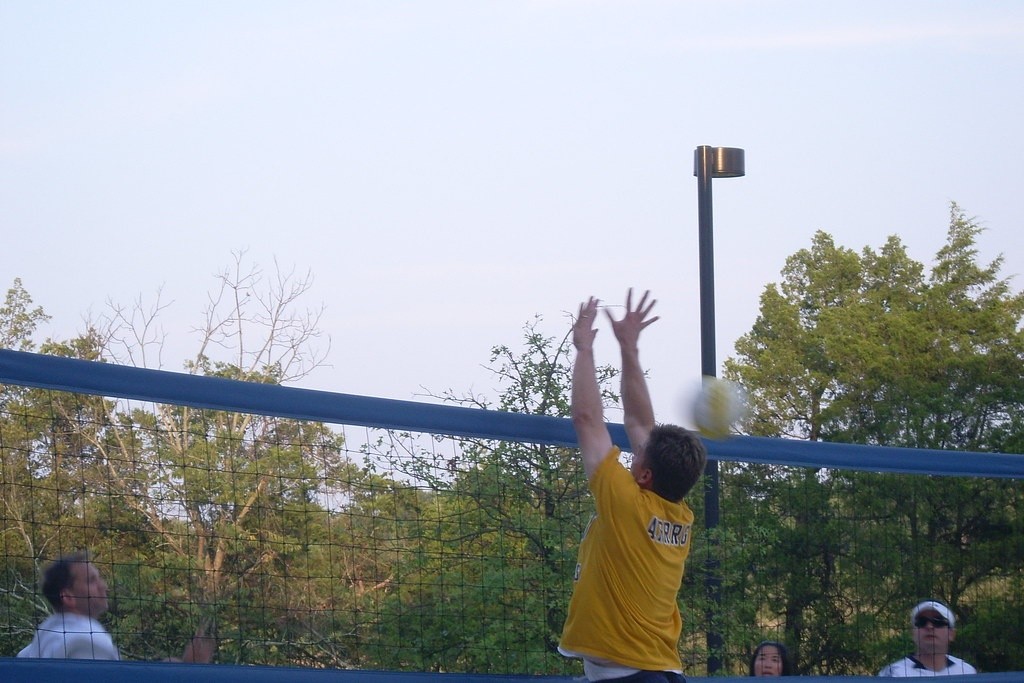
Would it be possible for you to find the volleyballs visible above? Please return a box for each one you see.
[691,380,740,438]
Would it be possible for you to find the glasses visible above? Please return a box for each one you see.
[914,615,951,629]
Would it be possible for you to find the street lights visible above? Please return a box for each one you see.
[693,145,746,678]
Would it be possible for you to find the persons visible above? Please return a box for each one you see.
[557,286,707,683]
[876,601,977,677]
[748,641,793,677]
[14,552,216,663]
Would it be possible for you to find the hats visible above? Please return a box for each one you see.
[909,598,955,631]
